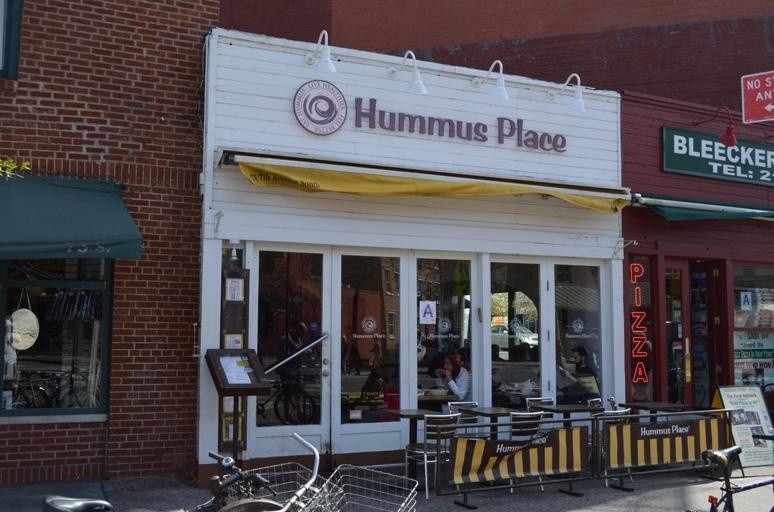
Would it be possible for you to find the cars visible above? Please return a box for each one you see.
[411,333,502,369]
[558,325,596,352]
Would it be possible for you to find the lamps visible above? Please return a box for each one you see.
[386,49,428,97]
[305,27,336,75]
[545,71,586,115]
[695,104,737,147]
[471,58,509,102]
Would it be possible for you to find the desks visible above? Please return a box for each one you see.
[618,401,689,423]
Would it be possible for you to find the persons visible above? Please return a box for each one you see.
[274,319,310,424]
[429,339,601,413]
[369,334,382,370]
[339,331,350,374]
[345,335,362,375]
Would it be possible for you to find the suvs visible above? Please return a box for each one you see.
[491,323,538,350]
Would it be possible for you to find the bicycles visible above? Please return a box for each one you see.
[40,450,345,511]
[679,432,773,511]
[257,431,418,512]
[633,356,774,408]
[10,365,90,408]
[252,364,319,425]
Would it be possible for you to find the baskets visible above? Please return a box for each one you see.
[222,461,419,512]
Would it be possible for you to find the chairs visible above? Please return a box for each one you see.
[379,395,633,498]
